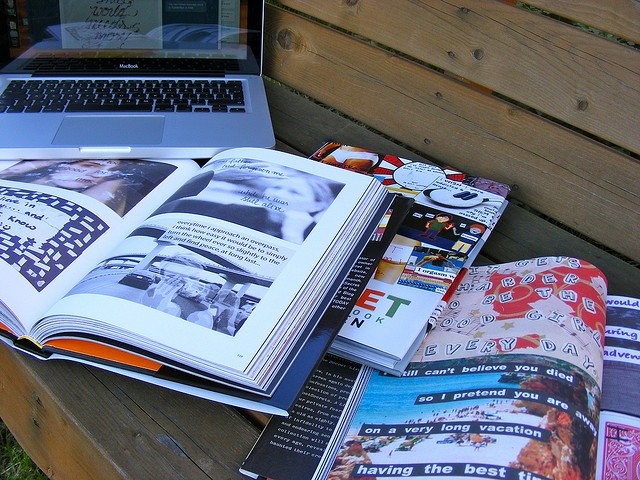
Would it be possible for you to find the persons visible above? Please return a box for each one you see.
[0,159,126,217]
[0,146,414,416]
[261,174,332,244]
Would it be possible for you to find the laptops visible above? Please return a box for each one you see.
[0,1,276,162]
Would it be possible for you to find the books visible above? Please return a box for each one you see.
[238,255,639,479]
[309,140,511,372]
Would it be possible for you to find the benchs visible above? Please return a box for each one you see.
[1,1,638,480]
